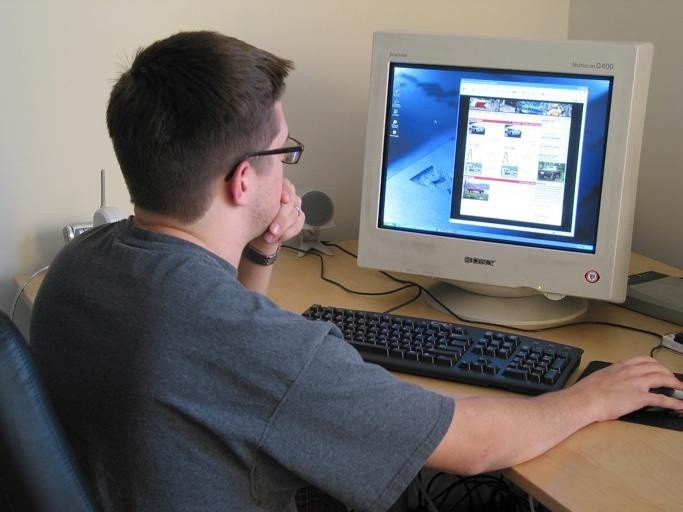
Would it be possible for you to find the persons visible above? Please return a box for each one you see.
[30,31,682,512]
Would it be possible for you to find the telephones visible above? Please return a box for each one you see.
[63,170,127,246]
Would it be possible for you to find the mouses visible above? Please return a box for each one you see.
[644,374,683,417]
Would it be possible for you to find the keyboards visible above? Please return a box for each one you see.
[295,303,585,395]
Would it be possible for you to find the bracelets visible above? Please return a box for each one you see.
[242,244,281,266]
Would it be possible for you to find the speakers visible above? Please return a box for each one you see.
[291,187,337,258]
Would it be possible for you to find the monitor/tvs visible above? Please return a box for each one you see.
[354,30,655,330]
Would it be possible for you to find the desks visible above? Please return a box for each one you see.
[12,241,682,511]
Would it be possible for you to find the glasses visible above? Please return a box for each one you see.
[224,137,304,181]
